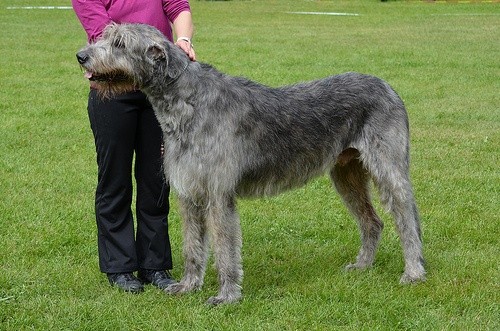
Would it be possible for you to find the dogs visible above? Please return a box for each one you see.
[75,23,426,308]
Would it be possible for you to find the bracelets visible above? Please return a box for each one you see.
[176,37,193,48]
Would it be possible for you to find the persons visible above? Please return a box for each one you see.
[72,0,195,294]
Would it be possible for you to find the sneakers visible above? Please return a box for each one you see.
[110,272,144,295]
[137,270,180,291]
